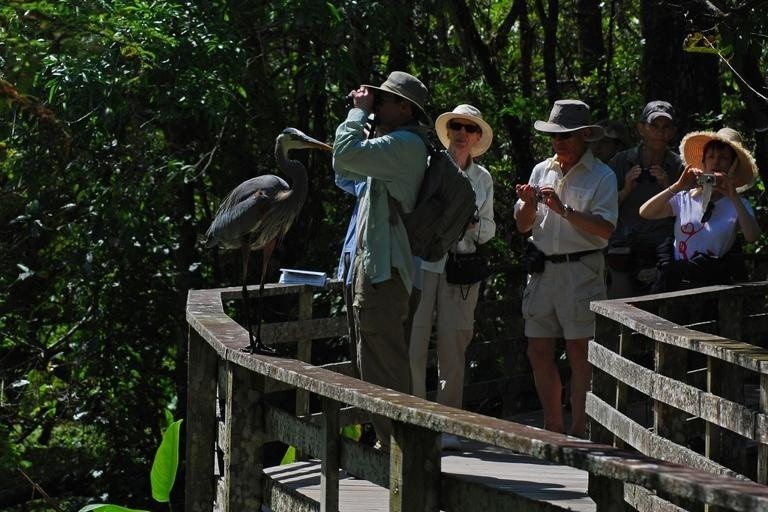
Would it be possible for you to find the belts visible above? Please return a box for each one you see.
[548,252,589,264]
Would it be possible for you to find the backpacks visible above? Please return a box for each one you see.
[385,125,476,263]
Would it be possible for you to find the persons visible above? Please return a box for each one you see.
[408,104,496,450]
[332,71,430,454]
[609,100,681,301]
[513,99,618,437]
[638,127,759,296]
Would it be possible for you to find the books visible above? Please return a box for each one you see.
[279,267,330,287]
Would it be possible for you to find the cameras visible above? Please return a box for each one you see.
[529,184,547,204]
[345,90,355,110]
[696,173,716,185]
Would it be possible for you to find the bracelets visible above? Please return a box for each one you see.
[668,186,677,195]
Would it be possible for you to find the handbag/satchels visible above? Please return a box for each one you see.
[445,238,490,284]
[662,253,707,292]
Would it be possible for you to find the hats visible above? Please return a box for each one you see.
[679,127,756,193]
[535,100,605,142]
[362,70,431,126]
[435,105,493,157]
[643,101,673,124]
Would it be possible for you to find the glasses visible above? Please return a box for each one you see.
[549,132,574,138]
[447,122,478,133]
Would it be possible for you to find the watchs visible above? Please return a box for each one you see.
[563,204,572,218]
[562,203,572,218]
